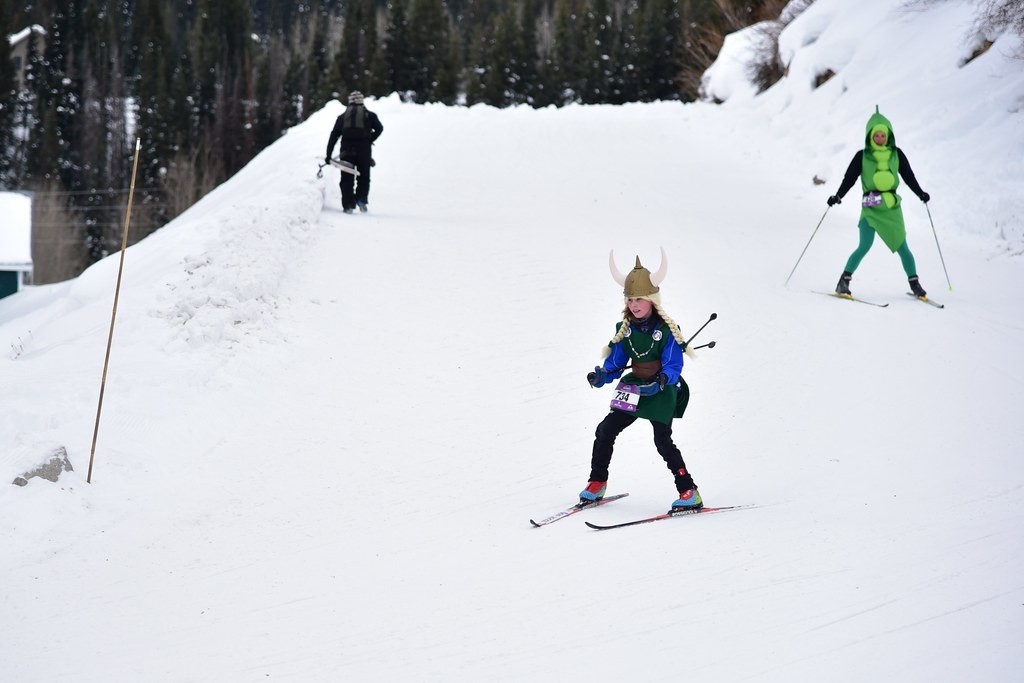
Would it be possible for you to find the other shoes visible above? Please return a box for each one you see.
[358,200,367,212]
[343,207,352,213]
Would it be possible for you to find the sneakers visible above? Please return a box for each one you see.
[835,276,852,295]
[909,276,926,296]
[579,481,607,501]
[671,489,704,510]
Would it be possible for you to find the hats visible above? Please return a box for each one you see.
[348,90,363,105]
[608,246,667,295]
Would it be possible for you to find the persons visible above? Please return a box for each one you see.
[324,91,383,214]
[827,105,931,297]
[578,268,703,514]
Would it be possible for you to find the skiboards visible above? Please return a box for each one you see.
[325,158,360,176]
[529,493,759,531]
[811,290,946,309]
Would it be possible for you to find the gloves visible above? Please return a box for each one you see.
[919,192,930,202]
[827,196,841,206]
[587,366,600,385]
[654,373,667,385]
[325,156,332,164]
